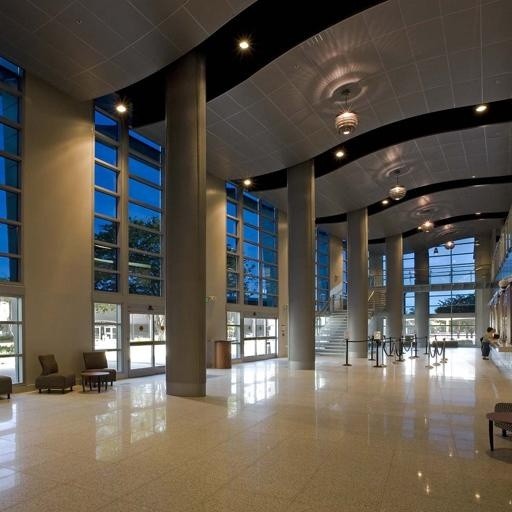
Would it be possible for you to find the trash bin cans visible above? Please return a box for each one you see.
[213,339,233,369]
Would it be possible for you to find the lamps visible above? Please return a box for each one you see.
[420,207,455,249]
[389,170,407,201]
[334,88,359,136]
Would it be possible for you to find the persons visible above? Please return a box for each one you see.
[480,327,493,360]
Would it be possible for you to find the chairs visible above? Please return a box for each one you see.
[0,351,117,400]
